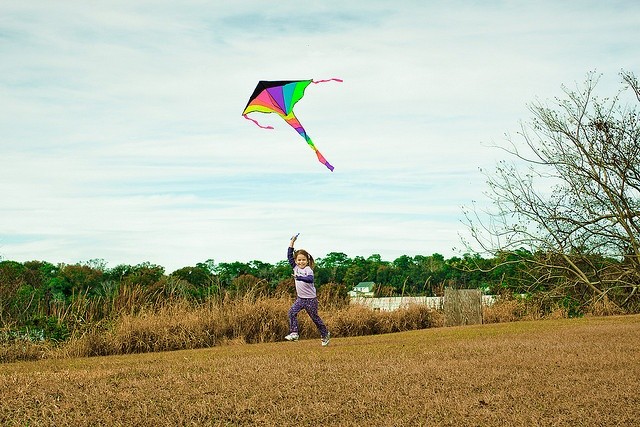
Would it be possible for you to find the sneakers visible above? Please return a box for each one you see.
[321,331,330,346]
[285,333,299,341]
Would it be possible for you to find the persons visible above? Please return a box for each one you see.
[284,235,331,347]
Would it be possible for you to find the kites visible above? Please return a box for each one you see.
[241,77,343,172]
